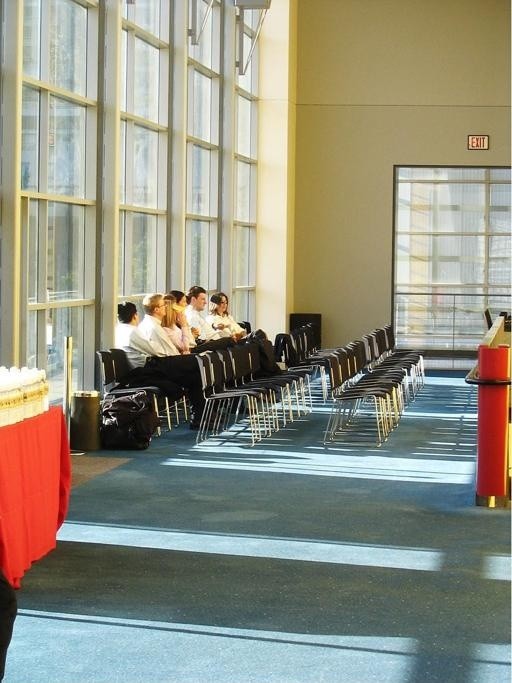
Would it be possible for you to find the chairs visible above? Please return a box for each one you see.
[194,324,344,447]
[322,323,426,446]
[98,347,188,438]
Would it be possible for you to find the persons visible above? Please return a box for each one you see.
[113,284,285,430]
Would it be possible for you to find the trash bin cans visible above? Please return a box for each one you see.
[70,391,99,451]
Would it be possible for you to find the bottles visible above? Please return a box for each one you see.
[0,365,49,428]
